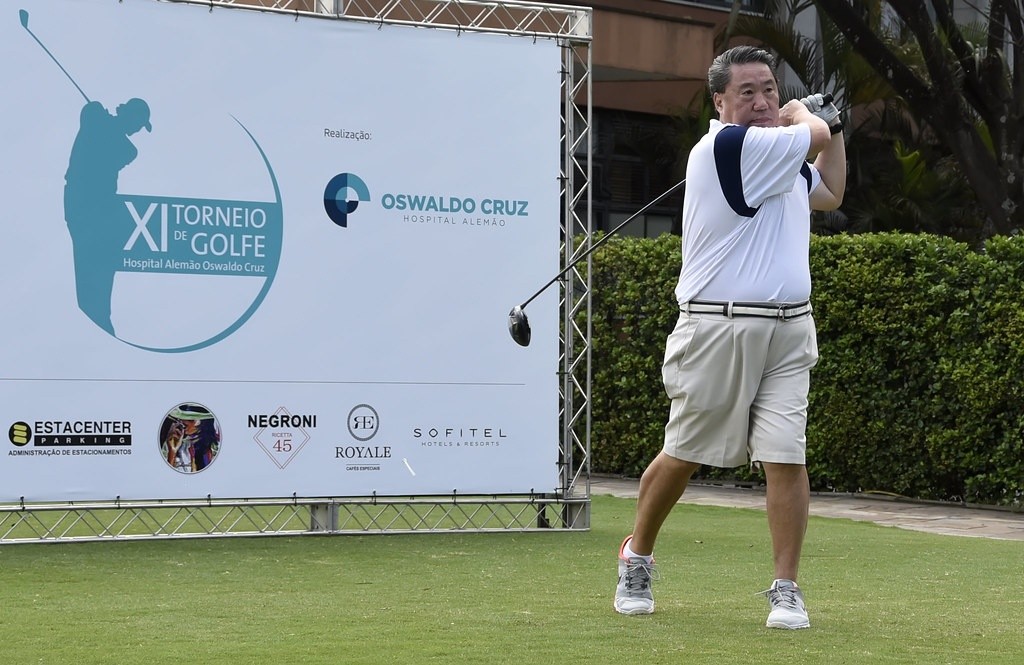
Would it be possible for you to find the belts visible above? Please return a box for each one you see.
[679,302,812,321]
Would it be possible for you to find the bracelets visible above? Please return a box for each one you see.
[829,121,843,136]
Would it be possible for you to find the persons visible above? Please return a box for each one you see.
[612,45,847,630]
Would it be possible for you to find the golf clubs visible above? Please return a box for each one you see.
[505,92,840,348]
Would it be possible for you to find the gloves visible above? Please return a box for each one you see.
[800,93,843,135]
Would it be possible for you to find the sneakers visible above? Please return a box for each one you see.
[613,534,660,615]
[755,579,810,630]
